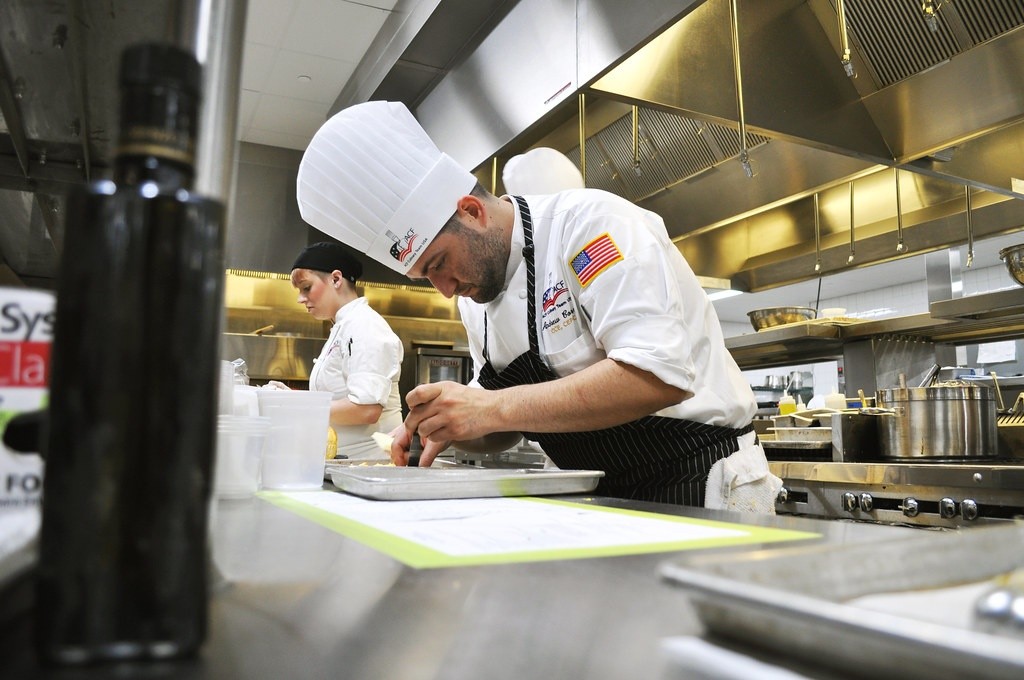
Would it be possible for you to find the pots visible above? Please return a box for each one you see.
[874,386,998,459]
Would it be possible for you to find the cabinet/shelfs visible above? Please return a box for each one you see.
[705,225,1024,532]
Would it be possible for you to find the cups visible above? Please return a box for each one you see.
[821,308,847,319]
[210,359,331,498]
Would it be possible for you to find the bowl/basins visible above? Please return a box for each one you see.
[1000,243,1024,286]
[747,306,818,332]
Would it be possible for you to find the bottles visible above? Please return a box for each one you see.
[35,39,226,662]
[779,390,807,416]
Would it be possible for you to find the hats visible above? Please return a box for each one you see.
[297,99,478,275]
[503,147,586,196]
[292,242,361,284]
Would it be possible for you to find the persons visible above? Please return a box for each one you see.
[261,241,405,460]
[293,96,777,521]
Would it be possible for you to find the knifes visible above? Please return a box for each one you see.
[408,382,426,466]
